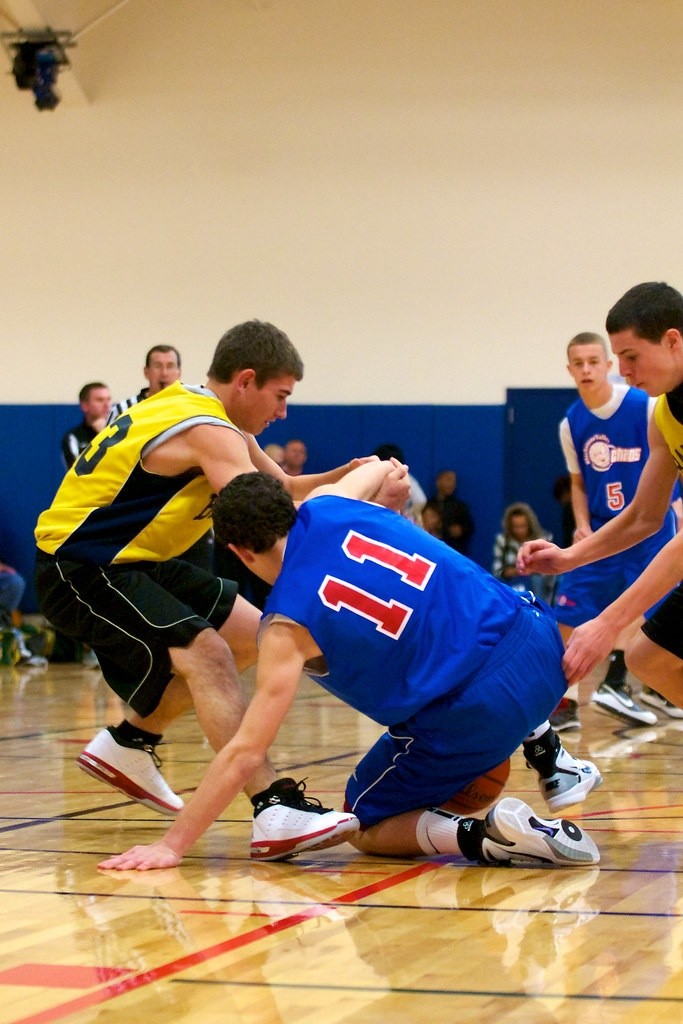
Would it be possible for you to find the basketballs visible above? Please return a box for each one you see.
[440,756,511,816]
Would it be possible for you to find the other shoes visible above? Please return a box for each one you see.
[81,650,100,670]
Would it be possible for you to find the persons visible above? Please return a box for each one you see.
[96,460,603,872]
[423,468,476,555]
[514,281,683,725]
[493,503,556,601]
[0,317,425,859]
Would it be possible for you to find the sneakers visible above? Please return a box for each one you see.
[74,866,180,921]
[76,725,184,816]
[478,798,600,866]
[556,730,582,755]
[590,682,657,725]
[640,702,683,732]
[538,733,603,813]
[589,726,657,771]
[549,697,581,732]
[249,860,358,921]
[481,865,600,940]
[250,776,361,861]
[639,683,683,719]
